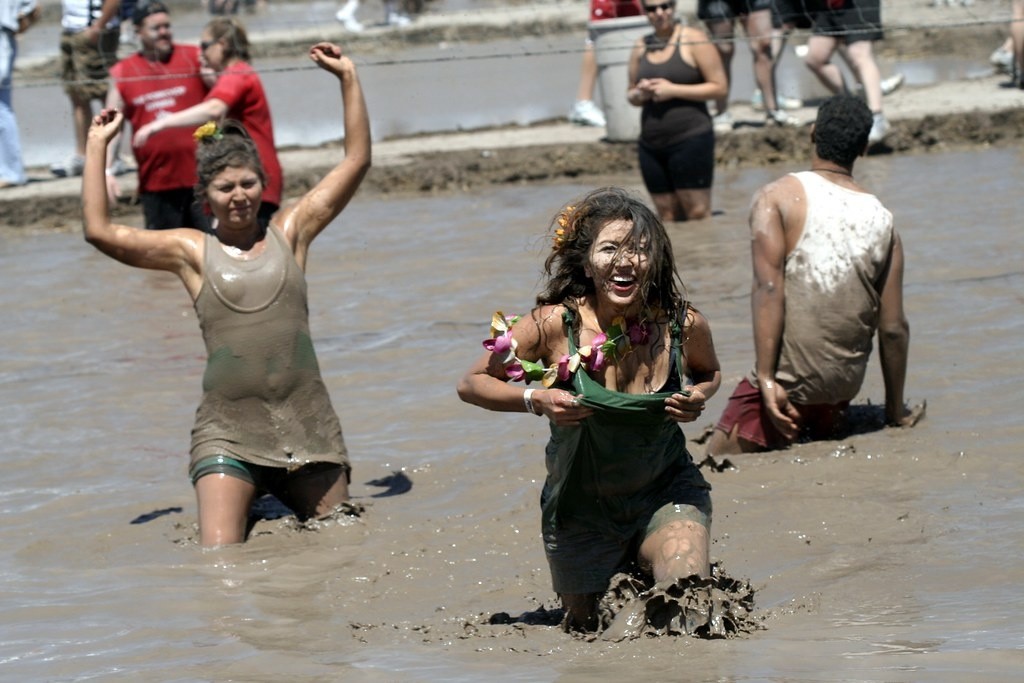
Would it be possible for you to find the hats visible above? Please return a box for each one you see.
[133,0,167,24]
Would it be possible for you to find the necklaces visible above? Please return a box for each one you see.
[809,165,851,177]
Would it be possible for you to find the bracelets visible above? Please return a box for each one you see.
[523,389,543,416]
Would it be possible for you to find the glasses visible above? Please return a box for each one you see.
[644,3,671,12]
[201,40,215,51]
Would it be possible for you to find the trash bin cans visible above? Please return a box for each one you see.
[588,14,683,142]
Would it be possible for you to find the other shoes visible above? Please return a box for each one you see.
[50,149,85,177]
[105,156,128,174]
[764,108,799,128]
[856,72,903,99]
[336,8,364,33]
[999,73,1024,89]
[990,47,1014,66]
[751,88,803,110]
[868,112,892,144]
[388,10,415,27]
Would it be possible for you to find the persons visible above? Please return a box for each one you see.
[456,186,722,634]
[0,0,1024,549]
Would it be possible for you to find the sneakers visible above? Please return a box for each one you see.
[569,99,606,127]
